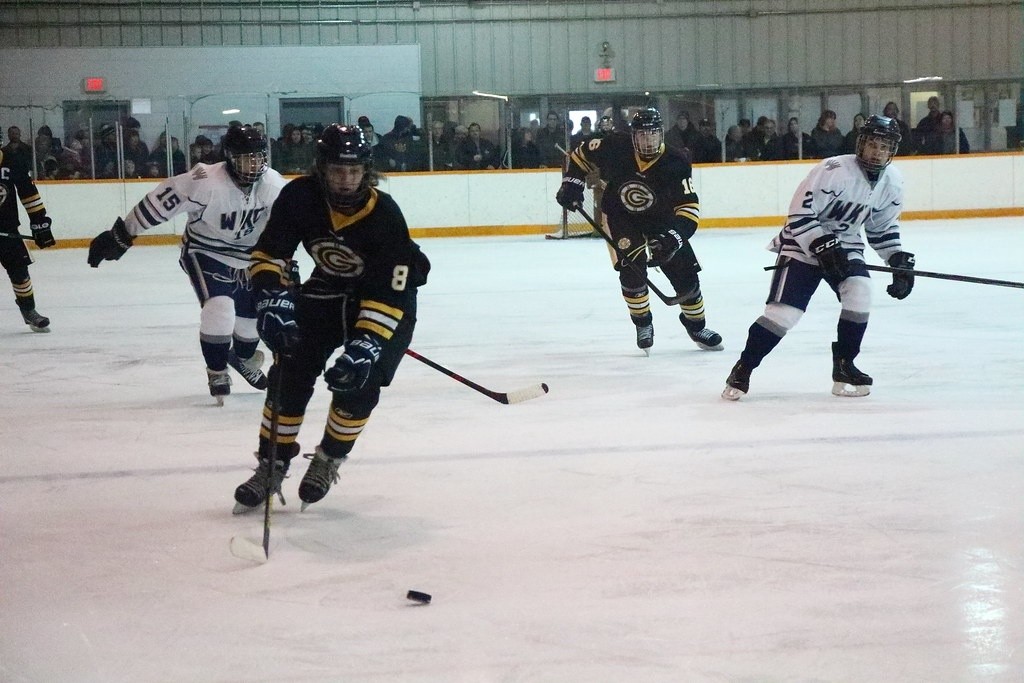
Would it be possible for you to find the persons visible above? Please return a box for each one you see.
[883,97,968,155]
[357,111,614,172]
[722,114,915,401]
[87,126,291,405]
[234,124,431,516]
[666,110,866,163]
[557,108,725,356]
[9,116,325,181]
[0,128,57,333]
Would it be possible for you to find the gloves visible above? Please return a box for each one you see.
[887,251,915,299]
[556,172,584,212]
[324,328,382,392]
[257,291,302,358]
[30,217,55,249]
[646,226,687,267]
[809,234,849,285]
[88,216,137,268]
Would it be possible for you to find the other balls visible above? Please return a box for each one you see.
[407,590,431,603]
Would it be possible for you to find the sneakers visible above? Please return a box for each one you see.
[298,446,348,512]
[206,366,232,406]
[832,342,873,396]
[20,308,51,333]
[630,311,653,357]
[723,351,758,400]
[226,347,268,391]
[680,313,724,350]
[233,436,300,513]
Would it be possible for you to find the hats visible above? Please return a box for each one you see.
[44,159,57,169]
[126,116,140,128]
[101,122,114,139]
[698,118,711,126]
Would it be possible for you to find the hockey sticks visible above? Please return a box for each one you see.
[554,142,607,189]
[846,262,1024,289]
[404,348,549,405]
[0,232,35,240]
[572,201,698,307]
[230,353,285,565]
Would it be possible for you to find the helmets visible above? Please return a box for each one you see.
[631,108,664,158]
[315,123,372,208]
[856,114,902,170]
[222,124,269,187]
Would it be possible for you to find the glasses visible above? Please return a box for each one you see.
[51,167,58,171]
[112,131,116,136]
